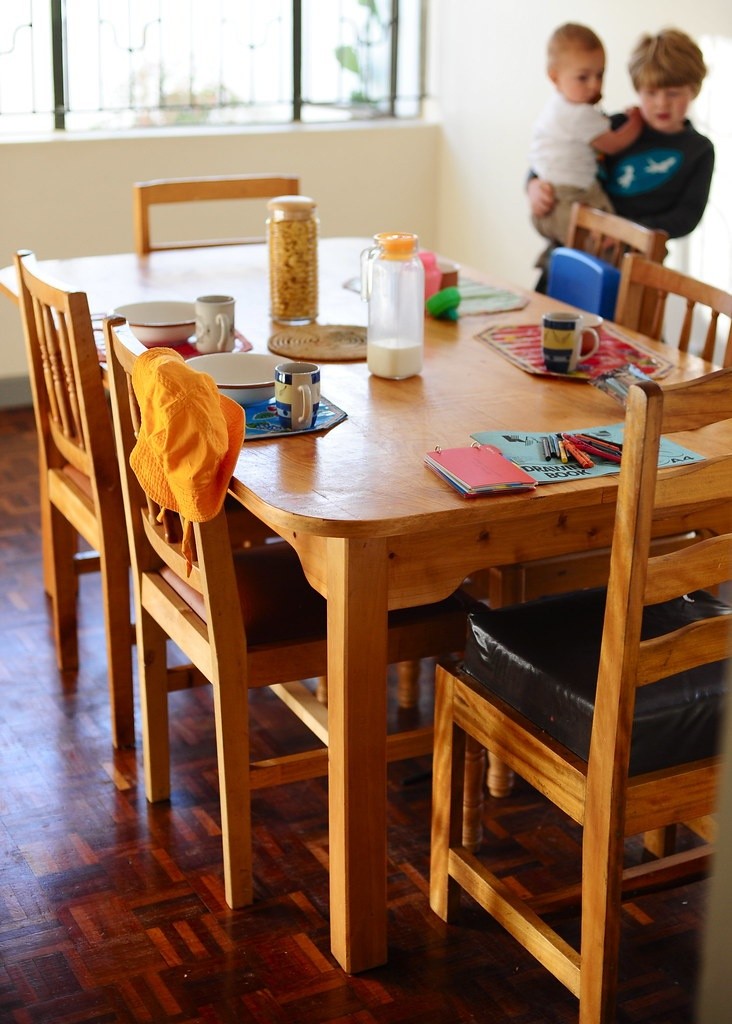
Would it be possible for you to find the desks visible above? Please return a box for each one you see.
[0,234,732,974]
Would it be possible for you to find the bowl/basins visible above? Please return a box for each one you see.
[581,313,604,356]
[184,351,294,407]
[112,300,196,348]
[436,261,458,291]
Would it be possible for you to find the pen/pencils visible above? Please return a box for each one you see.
[543,438,552,461]
[547,432,622,468]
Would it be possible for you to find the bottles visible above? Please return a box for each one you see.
[265,194,321,327]
[360,232,424,380]
[418,252,442,316]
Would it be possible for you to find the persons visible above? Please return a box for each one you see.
[526,24,715,322]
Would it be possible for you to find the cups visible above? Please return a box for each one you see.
[274,361,321,432]
[541,311,599,373]
[194,294,236,355]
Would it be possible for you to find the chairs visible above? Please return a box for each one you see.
[568,203,732,379]
[428,368,731,1023]
[135,178,299,251]
[17,248,328,747]
[98,316,495,914]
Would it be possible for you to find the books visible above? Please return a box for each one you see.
[421,440,539,499]
